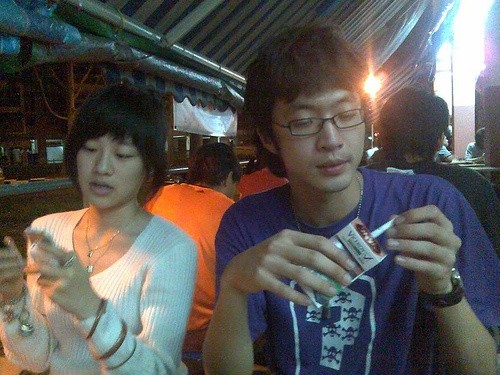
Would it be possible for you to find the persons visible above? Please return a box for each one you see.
[145,21,500,375]
[0,85,196,375]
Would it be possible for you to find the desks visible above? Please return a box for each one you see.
[440,159,500,172]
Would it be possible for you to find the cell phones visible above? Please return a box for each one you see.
[24,229,61,269]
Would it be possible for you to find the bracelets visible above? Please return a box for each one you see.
[92,318,129,361]
[0,282,34,337]
[86,299,108,341]
[107,337,137,369]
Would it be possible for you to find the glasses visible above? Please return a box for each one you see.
[271,108,373,136]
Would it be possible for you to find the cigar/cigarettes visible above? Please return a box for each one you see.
[370,218,396,239]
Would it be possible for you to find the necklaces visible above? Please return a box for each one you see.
[294,171,363,239]
[85,206,139,273]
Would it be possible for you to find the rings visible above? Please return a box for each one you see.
[64,255,75,268]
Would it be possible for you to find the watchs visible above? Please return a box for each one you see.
[417,266,465,307]
